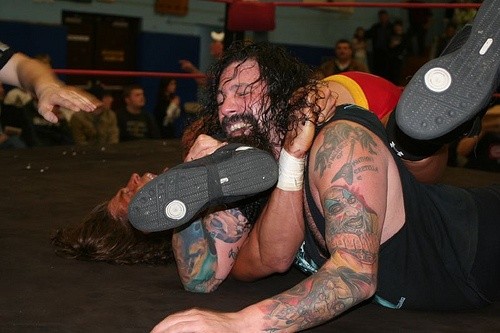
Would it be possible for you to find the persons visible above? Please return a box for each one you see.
[149,41,499,332]
[47,1,500,294]
[0,43,97,123]
[0,0,500,170]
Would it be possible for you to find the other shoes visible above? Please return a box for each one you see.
[127,143,280,233]
[395,0,500,141]
